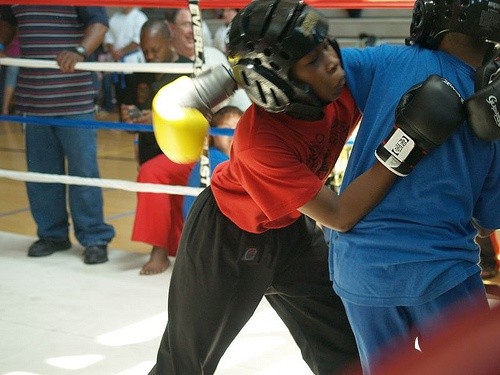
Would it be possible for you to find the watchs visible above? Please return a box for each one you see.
[69,45,87,58]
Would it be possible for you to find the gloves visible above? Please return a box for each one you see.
[373,74,466,179]
[150,64,238,166]
[467,51,500,141]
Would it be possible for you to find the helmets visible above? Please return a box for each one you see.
[406,1,500,50]
[225,0,344,121]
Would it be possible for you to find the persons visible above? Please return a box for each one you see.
[14,5,116,264]
[120,19,195,274]
[0,6,239,116]
[147,2,467,375]
[183,106,243,219]
[152,0,500,375]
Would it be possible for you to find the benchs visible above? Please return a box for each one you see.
[205,5,415,47]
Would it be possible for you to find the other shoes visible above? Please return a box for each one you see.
[26,240,72,258]
[82,245,109,264]
